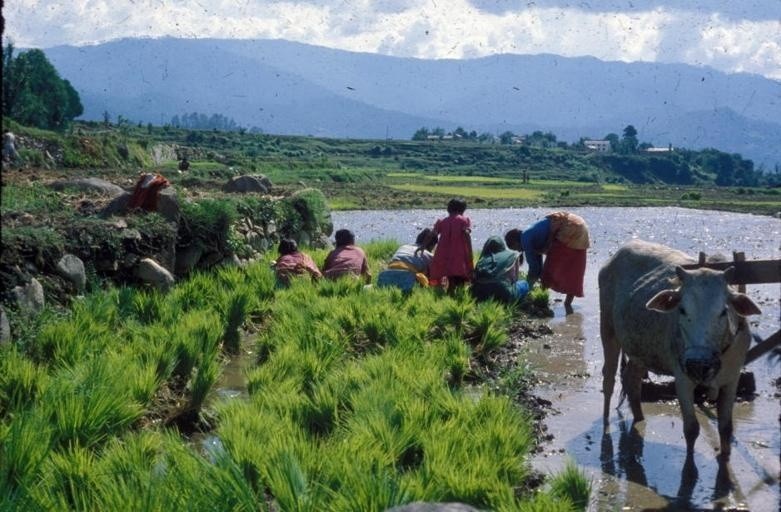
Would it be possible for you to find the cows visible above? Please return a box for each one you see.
[597,246,762,492]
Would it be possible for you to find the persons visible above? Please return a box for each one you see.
[471,234,531,302]
[1,127,18,163]
[505,209,591,315]
[414,197,475,299]
[269,237,323,293]
[376,227,440,297]
[320,227,372,286]
